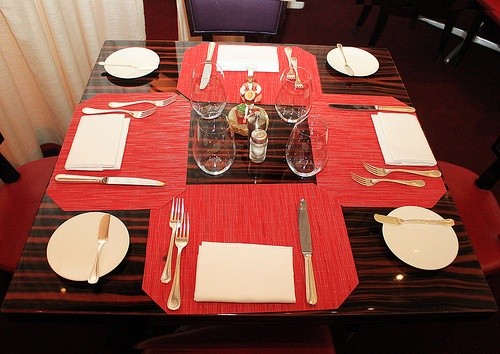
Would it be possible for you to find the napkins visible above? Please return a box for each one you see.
[194,242,297,303]
[371,112,437,167]
[64,114,130,171]
[217,45,279,73]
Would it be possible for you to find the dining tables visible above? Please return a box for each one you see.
[436,140,500,276]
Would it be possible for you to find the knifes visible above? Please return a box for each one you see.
[373,213,455,226]
[97,60,158,71]
[298,198,319,305]
[199,41,216,90]
[88,214,111,285]
[338,43,353,76]
[53,173,166,188]
[329,103,416,114]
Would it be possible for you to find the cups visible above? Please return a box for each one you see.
[193,111,236,175]
[191,62,228,119]
[275,66,313,124]
[285,111,330,177]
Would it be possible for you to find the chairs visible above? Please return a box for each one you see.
[0,132,59,273]
[184,0,288,42]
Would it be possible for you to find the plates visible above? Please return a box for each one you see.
[104,47,160,79]
[46,211,130,281]
[382,205,459,270]
[326,46,379,77]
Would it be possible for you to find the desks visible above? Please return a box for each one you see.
[0,40,500,354]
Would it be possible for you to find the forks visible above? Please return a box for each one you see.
[107,94,181,109]
[81,107,158,118]
[167,212,190,310]
[160,197,186,283]
[283,47,305,90]
[362,160,442,178]
[350,172,425,188]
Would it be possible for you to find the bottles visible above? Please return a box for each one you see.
[247,112,269,162]
